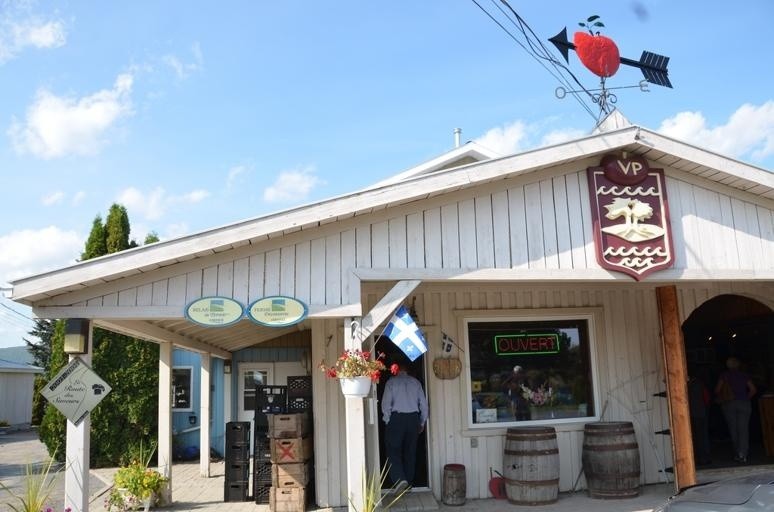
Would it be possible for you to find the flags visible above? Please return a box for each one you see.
[380,304,429,364]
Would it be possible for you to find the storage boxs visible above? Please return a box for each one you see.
[268,486,311,512]
[268,437,314,463]
[270,461,314,487]
[222,420,252,504]
[265,412,311,439]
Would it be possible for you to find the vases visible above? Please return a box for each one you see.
[117,487,160,511]
[336,375,372,398]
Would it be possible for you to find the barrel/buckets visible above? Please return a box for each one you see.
[582,422,640,499]
[443,464,466,506]
[504,425,560,505]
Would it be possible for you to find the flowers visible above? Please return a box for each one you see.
[103,460,169,511]
[520,380,553,407]
[317,348,399,383]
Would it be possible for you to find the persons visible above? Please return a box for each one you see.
[715,354,756,465]
[501,364,535,420]
[380,352,427,496]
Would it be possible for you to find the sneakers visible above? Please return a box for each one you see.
[732,453,747,462]
[393,478,414,499]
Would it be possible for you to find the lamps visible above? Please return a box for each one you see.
[62,316,89,356]
[222,359,233,375]
